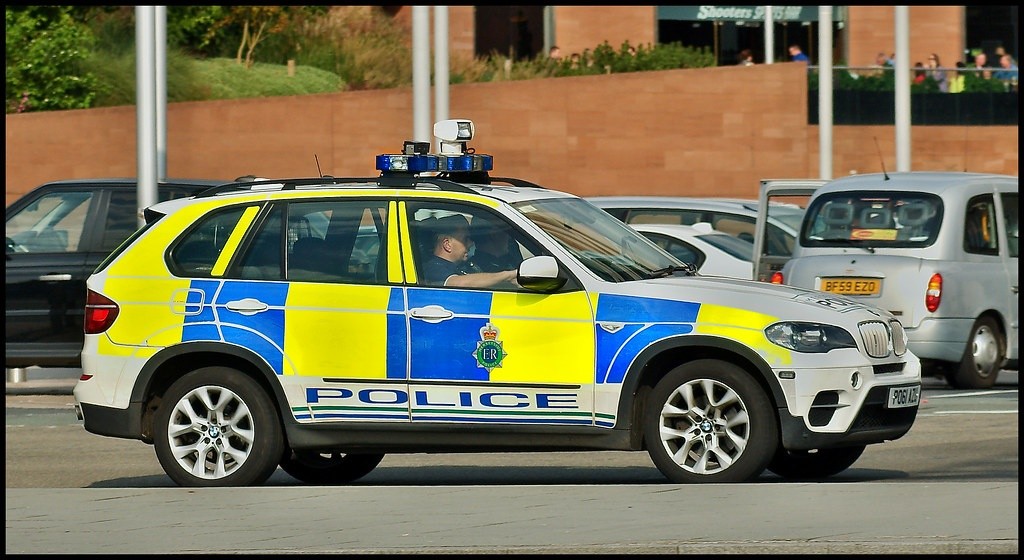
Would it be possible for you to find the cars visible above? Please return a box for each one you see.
[578,194,798,253]
[623,218,758,281]
[71,117,925,484]
[749,138,1019,389]
[3,178,235,370]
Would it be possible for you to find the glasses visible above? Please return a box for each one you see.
[926,57,936,61]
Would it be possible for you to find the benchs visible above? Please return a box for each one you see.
[239,238,348,280]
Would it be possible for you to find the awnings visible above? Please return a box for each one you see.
[656,3,849,27]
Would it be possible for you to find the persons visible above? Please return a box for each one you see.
[737,46,757,71]
[859,34,1019,114]
[789,45,809,69]
[461,209,565,289]
[491,34,660,88]
[420,213,544,293]
